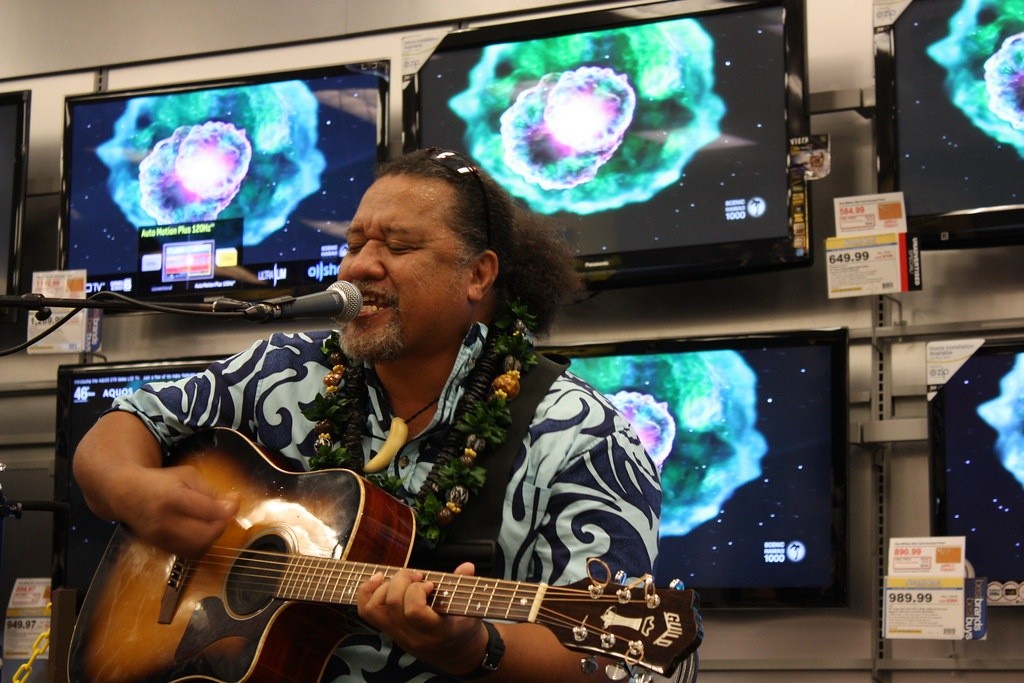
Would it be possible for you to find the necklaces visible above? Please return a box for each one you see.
[304,304,533,559]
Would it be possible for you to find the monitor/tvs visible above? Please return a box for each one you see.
[59,59,392,314]
[927,334,1023,607]
[53,354,235,617]
[399,0,815,292]
[872,0,1024,250]
[532,327,850,609]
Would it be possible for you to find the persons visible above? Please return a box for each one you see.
[73,149,667,683]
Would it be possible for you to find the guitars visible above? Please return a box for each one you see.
[64,424,706,683]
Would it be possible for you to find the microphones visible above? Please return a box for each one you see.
[244,280,363,323]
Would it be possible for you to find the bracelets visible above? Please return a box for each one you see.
[440,621,506,683]
